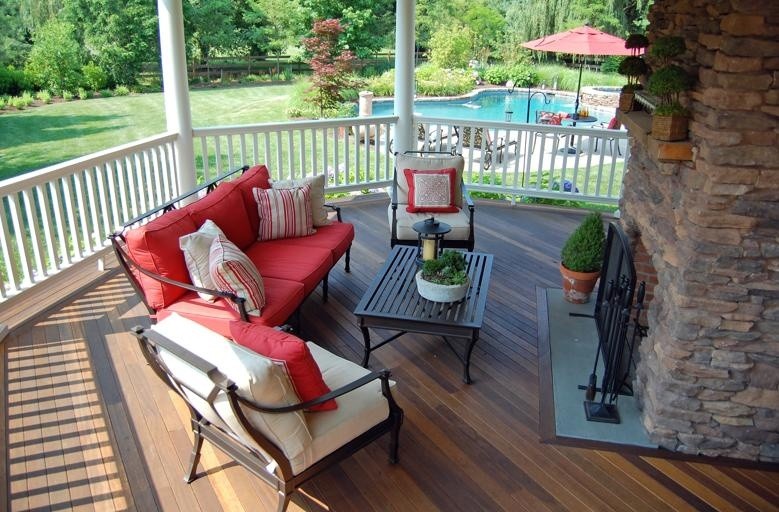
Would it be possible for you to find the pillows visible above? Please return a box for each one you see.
[269,175,334,226]
[402,168,458,214]
[609,119,621,129]
[540,110,562,125]
[228,320,339,413]
[179,219,226,304]
[210,234,267,318]
[253,183,318,242]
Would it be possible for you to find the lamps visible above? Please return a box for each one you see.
[412,217,452,267]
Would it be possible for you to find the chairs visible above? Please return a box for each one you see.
[387,112,457,157]
[592,108,623,156]
[450,123,517,170]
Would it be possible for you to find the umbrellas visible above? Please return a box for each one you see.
[519,25,645,154]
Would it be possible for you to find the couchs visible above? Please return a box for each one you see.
[130,310,406,511]
[387,150,475,252]
[108,164,355,336]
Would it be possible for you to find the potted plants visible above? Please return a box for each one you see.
[560,212,608,305]
[415,249,472,302]
[618,34,648,112]
[649,35,696,142]
